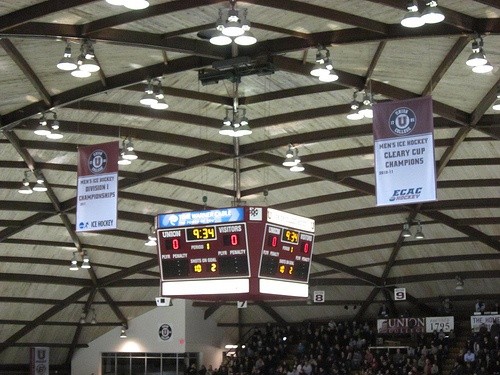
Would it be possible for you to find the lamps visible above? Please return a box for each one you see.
[34,110,63,140]
[281,142,306,173]
[493,92,500,110]
[347,88,375,121]
[209,0,258,47]
[118,135,141,166]
[145,226,158,247]
[79,307,97,325]
[465,33,493,75]
[119,323,127,338]
[69,249,91,271]
[308,43,340,83]
[455,274,465,290]
[18,168,48,195]
[140,76,169,110]
[400,211,425,239]
[218,107,253,138]
[306,295,313,304]
[55,37,102,80]
[400,0,446,28]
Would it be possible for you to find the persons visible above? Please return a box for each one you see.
[450,320,500,375]
[472,297,485,315]
[378,303,391,319]
[184,320,455,375]
[493,302,500,314]
[440,298,453,316]
